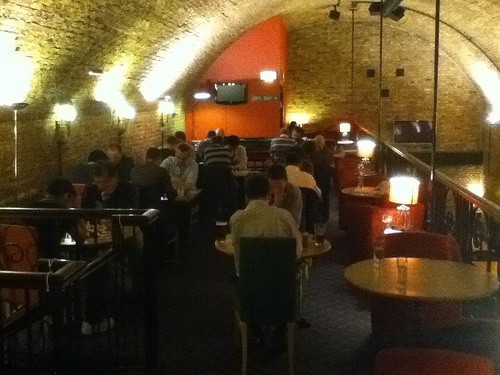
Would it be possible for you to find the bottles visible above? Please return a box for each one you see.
[372,237,385,274]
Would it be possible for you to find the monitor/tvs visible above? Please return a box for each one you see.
[214,82,247,105]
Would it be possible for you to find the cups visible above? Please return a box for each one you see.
[313,222,326,244]
[216,221,227,243]
[397,238,408,268]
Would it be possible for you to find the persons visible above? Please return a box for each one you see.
[161,143,199,238]
[224,135,247,171]
[199,135,240,171]
[73,151,109,186]
[128,147,178,216]
[261,166,304,232]
[229,174,311,328]
[107,145,135,180]
[18,178,105,312]
[82,160,160,306]
[269,121,336,189]
[160,127,224,156]
[283,147,322,199]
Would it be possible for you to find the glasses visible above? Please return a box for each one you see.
[92,178,111,186]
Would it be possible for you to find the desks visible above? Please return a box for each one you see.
[345,256,500,334]
[216,231,331,321]
[61,220,140,249]
[231,168,261,207]
[158,188,207,259]
[339,187,423,229]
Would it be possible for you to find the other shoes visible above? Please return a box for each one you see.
[81,317,115,336]
[247,334,260,348]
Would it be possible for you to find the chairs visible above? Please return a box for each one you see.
[0,139,495,375]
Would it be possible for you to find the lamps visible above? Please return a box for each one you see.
[388,176,420,211]
[339,123,351,136]
[357,141,376,160]
